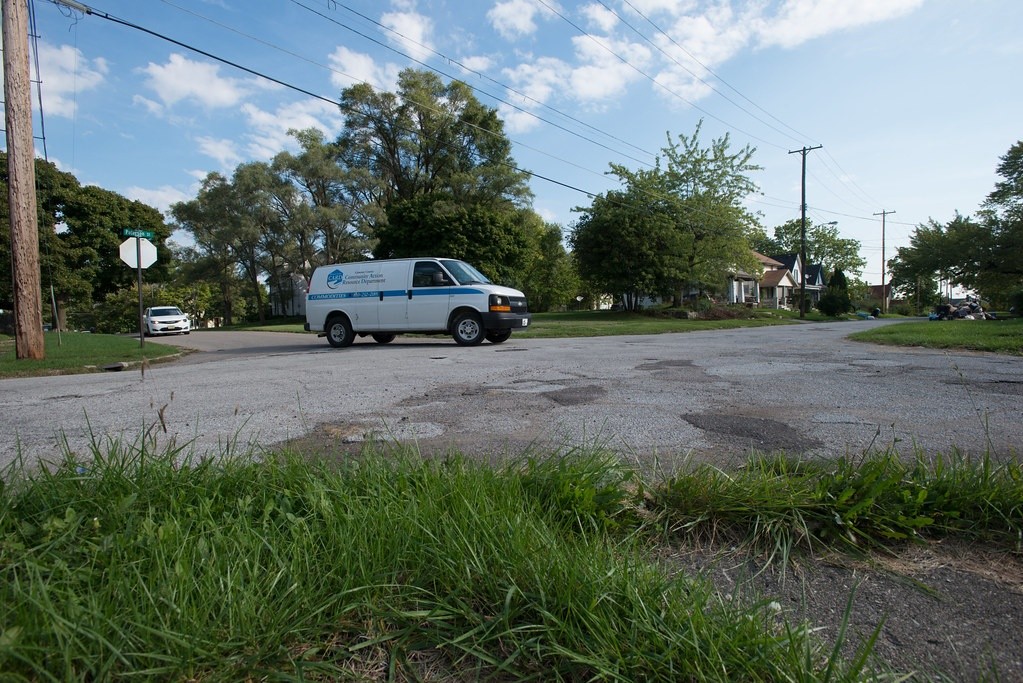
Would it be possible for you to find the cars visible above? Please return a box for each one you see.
[142,306,191,337]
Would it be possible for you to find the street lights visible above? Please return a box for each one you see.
[806,220,839,232]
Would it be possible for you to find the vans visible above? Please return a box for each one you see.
[304,257,533,348]
